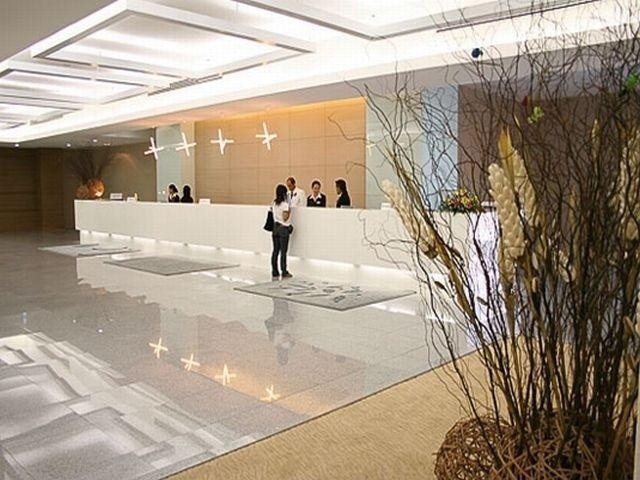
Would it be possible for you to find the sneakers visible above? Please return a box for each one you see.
[272,272,292,278]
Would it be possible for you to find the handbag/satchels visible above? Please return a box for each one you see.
[263,210,275,231]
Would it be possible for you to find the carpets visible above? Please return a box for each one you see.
[233,275,418,313]
[37,241,240,277]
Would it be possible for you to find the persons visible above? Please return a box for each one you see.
[285,177,327,207]
[264,185,293,277]
[168,184,193,202]
[265,298,297,365]
[335,180,350,208]
